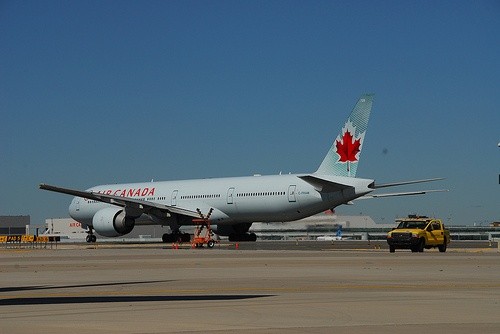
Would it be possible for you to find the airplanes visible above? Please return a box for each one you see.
[39,93,449,243]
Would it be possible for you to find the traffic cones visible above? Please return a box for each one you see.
[172,244,174,250]
[236,243,239,250]
[176,244,178,249]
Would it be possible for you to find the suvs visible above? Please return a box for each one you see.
[386,215,450,252]
